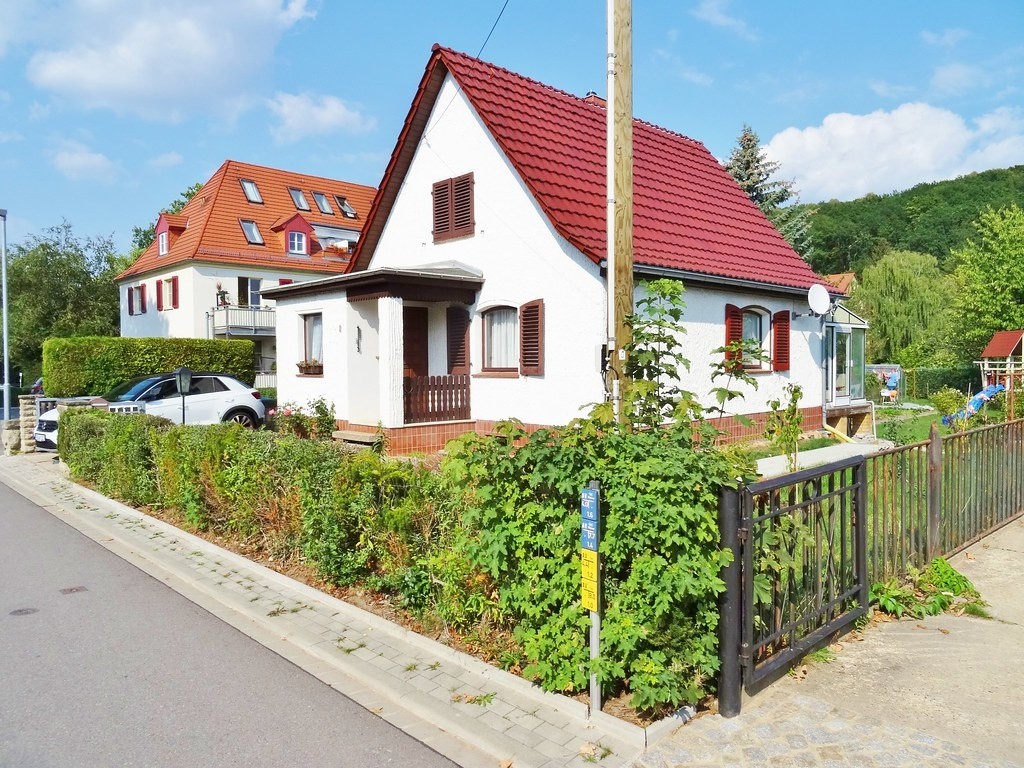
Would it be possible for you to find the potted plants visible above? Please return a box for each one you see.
[296,358,323,374]
[220,297,226,305]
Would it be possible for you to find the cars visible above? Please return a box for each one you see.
[30,376,44,394]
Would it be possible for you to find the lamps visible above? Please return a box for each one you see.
[172,366,193,395]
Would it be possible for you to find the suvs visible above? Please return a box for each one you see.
[32,372,265,449]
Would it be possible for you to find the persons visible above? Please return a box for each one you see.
[886,369,897,401]
